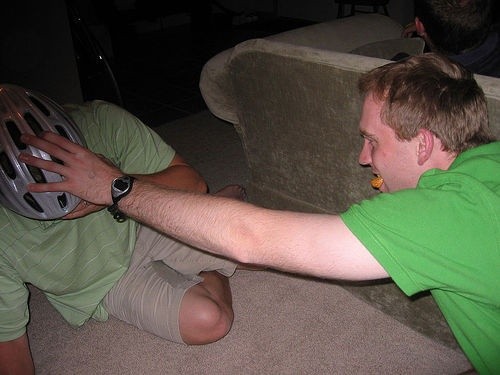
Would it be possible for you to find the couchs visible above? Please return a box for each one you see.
[199,12,500,352]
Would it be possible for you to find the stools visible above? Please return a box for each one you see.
[334,0,393,19]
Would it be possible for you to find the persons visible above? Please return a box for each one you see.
[0,78,269,374]
[400,0,500,79]
[16,53,499,374]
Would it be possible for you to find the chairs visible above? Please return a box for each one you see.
[63,0,127,108]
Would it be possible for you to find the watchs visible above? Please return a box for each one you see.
[106,174,138,221]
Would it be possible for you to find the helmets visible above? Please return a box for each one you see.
[0,83,89,220]
